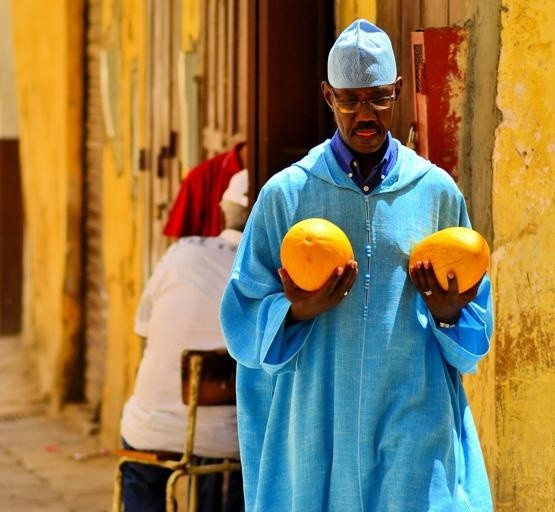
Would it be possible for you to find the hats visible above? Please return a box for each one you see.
[327,18,399,91]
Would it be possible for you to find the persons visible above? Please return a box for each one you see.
[218,18,495,512]
[163,141,248,238]
[118,170,251,512]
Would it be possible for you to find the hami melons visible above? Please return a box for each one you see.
[410,226,490,296]
[279,218,357,290]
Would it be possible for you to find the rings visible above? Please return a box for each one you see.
[343,291,347,296]
[424,290,431,296]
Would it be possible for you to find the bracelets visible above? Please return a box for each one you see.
[439,315,461,328]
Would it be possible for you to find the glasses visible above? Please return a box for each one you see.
[330,84,398,115]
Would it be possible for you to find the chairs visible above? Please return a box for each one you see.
[111,348,241,512]
